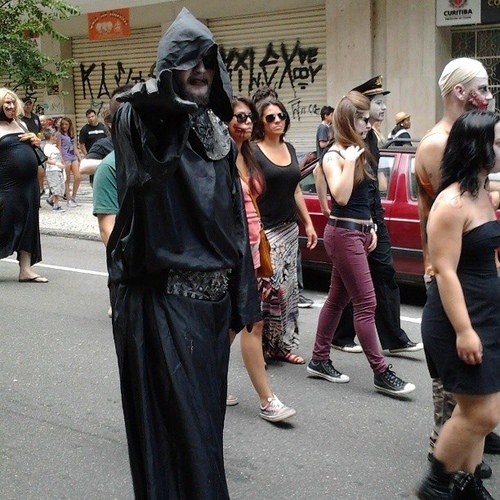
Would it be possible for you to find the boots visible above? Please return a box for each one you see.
[456,460,496,500]
[418,453,456,500]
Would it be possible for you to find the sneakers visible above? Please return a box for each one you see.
[259,393,297,422]
[53,206,65,212]
[46,198,54,207]
[375,367,415,395]
[226,393,237,406]
[307,358,349,383]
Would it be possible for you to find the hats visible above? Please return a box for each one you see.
[395,112,411,125]
[23,99,32,104]
[351,75,391,97]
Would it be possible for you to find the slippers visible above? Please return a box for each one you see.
[18,274,49,283]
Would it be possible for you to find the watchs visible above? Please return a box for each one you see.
[369,223,377,232]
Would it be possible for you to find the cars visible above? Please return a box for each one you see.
[293,137,500,292]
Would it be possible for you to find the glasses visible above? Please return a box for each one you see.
[232,113,256,123]
[265,113,288,122]
[358,116,370,122]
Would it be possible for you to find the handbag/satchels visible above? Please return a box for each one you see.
[17,122,48,166]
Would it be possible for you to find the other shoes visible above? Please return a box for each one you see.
[71,197,82,206]
[484,432,500,453]
[389,341,425,353]
[330,343,363,353]
[476,461,493,478]
[296,295,314,308]
[67,199,76,207]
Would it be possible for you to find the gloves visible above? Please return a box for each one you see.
[117,69,197,128]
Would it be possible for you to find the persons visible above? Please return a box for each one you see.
[415,57,500,478]
[36,114,66,214]
[92,84,137,250]
[57,117,83,207]
[105,6,264,500]
[79,108,108,190]
[217,94,299,424]
[0,86,50,285]
[415,109,500,500]
[252,97,318,369]
[20,97,42,136]
[315,105,336,157]
[79,108,138,318]
[305,89,419,397]
[388,112,413,147]
[328,74,425,355]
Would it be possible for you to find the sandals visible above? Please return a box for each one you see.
[278,352,305,364]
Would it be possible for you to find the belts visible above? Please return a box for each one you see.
[327,219,378,233]
